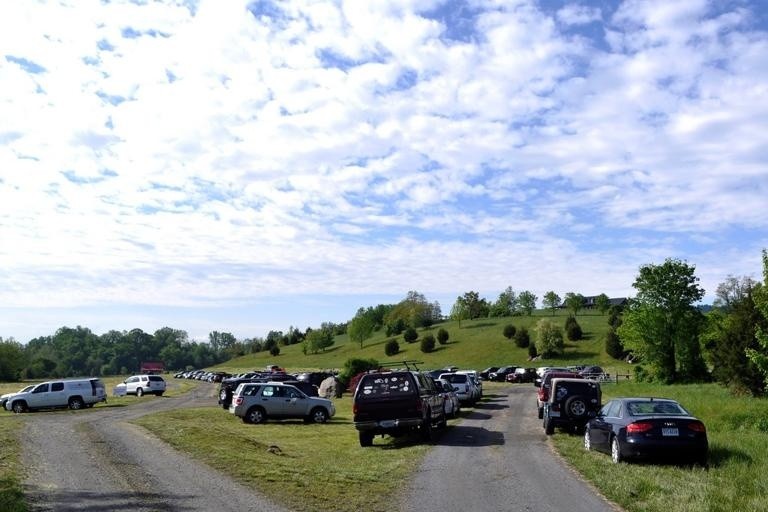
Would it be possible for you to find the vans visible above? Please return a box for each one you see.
[0,377,108,413]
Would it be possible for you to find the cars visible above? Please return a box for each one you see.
[583,397,709,464]
[173,370,321,386]
[479,364,609,384]
[116,375,166,397]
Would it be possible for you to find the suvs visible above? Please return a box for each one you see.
[218,364,337,424]
[534,372,602,435]
[353,361,484,446]
